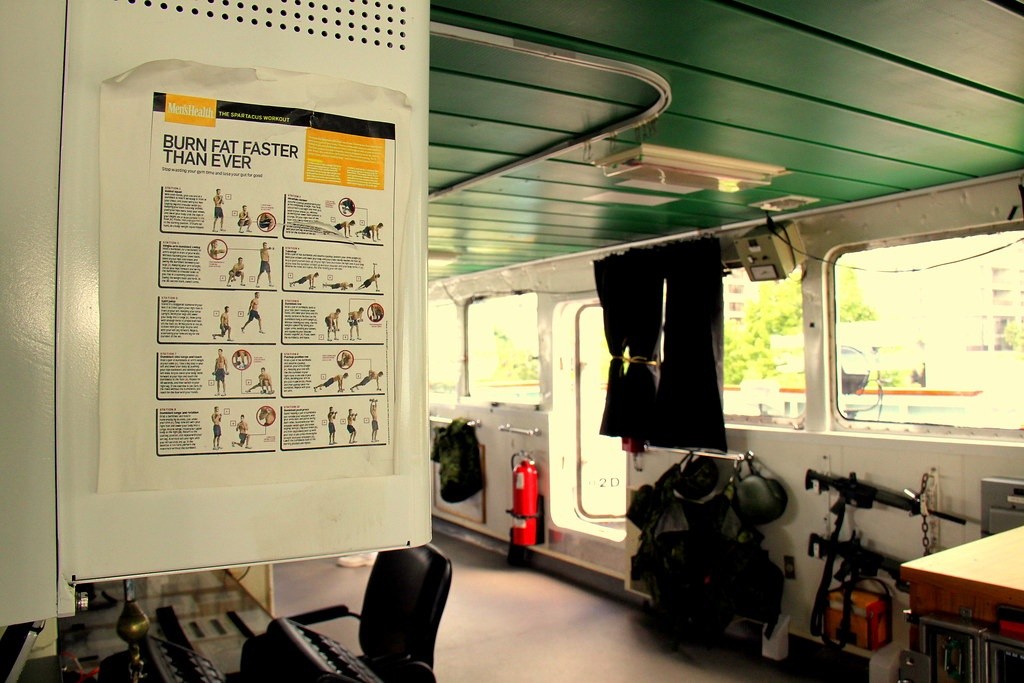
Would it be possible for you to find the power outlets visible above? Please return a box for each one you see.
[784,555,796,580]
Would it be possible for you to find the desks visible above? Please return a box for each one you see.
[56,568,321,683]
[897,525,1024,683]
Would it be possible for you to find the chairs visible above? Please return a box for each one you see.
[286,542,453,683]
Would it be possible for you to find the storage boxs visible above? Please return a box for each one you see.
[822,585,893,651]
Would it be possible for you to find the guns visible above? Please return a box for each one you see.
[804,467,968,526]
[807,528,909,584]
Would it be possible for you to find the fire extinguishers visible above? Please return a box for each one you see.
[510,450,539,545]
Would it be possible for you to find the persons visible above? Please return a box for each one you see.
[232,415,252,449]
[213,349,228,396]
[256,242,274,288]
[226,257,245,288]
[347,409,358,443]
[260,407,274,424]
[210,241,225,258]
[248,367,273,391]
[328,407,338,445]
[289,272,319,289]
[241,292,264,334]
[324,309,340,341]
[370,399,379,442]
[347,308,364,341]
[355,265,380,291]
[212,306,233,341]
[260,214,274,231]
[212,407,224,450]
[313,373,348,392]
[212,188,226,232]
[238,205,252,233]
[323,282,353,290]
[354,223,383,242]
[350,369,383,392]
[323,220,355,238]
